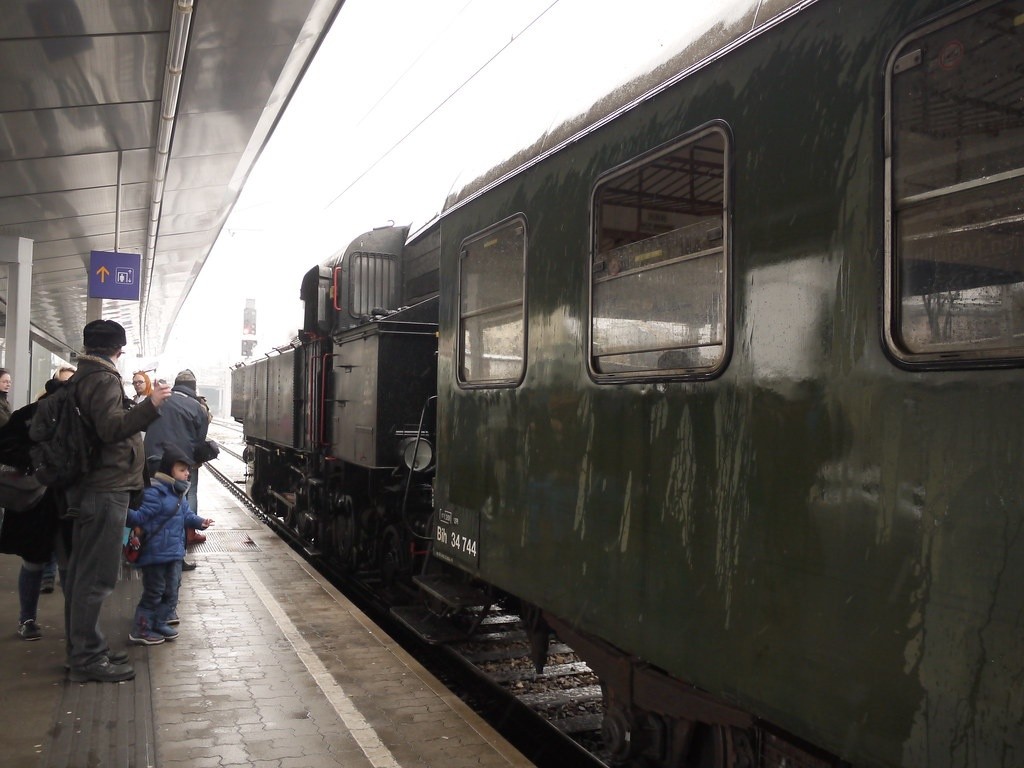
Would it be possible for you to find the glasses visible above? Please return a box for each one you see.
[0,381,12,384]
[132,380,146,385]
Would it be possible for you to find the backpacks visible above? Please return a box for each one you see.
[25,367,138,491]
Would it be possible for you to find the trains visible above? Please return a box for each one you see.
[231,0,1024,768]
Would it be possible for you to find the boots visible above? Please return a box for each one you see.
[186,527,206,544]
[129,604,179,644]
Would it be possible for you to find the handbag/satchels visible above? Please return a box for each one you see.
[123,526,144,565]
[0,465,58,512]
[193,441,219,464]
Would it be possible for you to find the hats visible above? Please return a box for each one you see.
[84,319,126,347]
[175,369,196,391]
[155,442,194,477]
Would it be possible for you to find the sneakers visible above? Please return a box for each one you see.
[40,576,55,592]
[165,606,180,624]
[17,619,42,640]
[64,649,136,682]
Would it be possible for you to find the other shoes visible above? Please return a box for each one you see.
[182,559,196,570]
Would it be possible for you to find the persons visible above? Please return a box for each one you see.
[129,370,154,442]
[65,320,171,682]
[141,370,210,573]
[0,364,76,641]
[0,367,13,429]
[125,451,215,644]
[183,466,210,545]
